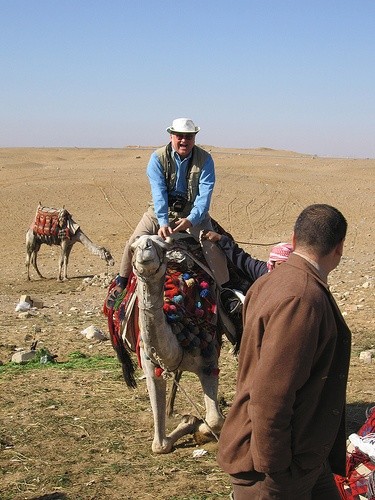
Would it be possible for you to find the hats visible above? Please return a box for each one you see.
[166,118,201,135]
[267,243,293,272]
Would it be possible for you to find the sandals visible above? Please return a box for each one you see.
[106,283,123,310]
[219,288,246,314]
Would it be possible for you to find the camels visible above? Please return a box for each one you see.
[25,205,115,283]
[130,235,226,454]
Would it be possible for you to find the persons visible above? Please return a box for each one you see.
[205,230,294,281]
[215,204,351,500]
[105,118,241,314]
